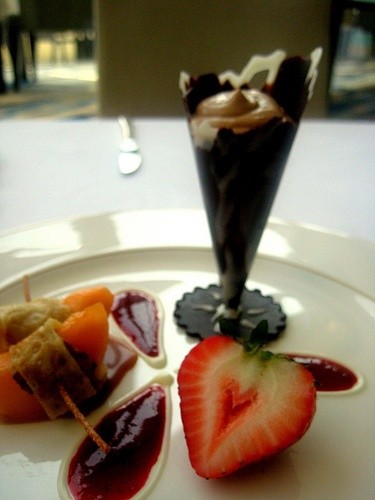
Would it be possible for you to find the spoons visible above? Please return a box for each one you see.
[112,115,143,177]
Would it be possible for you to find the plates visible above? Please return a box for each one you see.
[0,205,375,500]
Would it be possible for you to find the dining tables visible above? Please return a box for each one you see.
[4,115,374,248]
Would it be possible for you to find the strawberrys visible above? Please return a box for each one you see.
[176,318,316,479]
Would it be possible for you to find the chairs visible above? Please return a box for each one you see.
[95,0,329,118]
